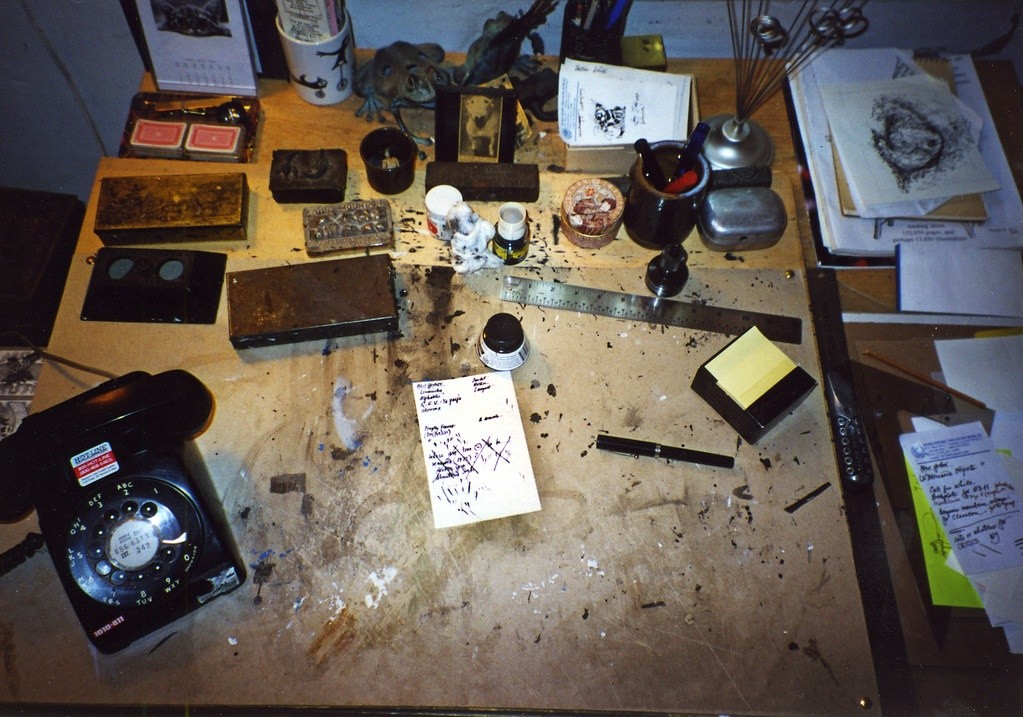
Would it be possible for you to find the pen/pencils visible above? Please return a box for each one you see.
[595,434,735,468]
[571,0,629,31]
[633,121,711,194]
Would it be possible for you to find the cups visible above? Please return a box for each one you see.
[558,0,628,74]
[275,11,356,105]
[360,127,417,195]
[624,140,711,251]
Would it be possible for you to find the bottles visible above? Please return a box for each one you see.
[476,313,531,372]
[492,201,530,265]
[425,185,463,241]
[645,243,689,297]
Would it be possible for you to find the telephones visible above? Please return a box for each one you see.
[0,369,247,655]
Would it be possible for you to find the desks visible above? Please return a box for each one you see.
[1,55,1023,716]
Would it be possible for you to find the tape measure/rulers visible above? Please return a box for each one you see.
[497,273,803,345]
